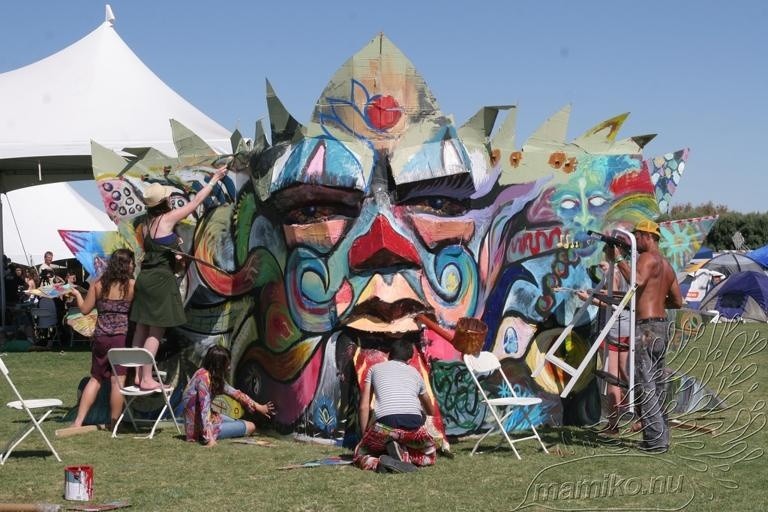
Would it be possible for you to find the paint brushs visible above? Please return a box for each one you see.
[225,156,233,166]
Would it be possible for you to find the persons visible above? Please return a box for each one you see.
[23,267,38,302]
[14,266,22,276]
[605,219,682,453]
[69,248,136,426]
[63,272,78,308]
[129,164,228,391]
[353,339,436,475]
[182,346,277,449]
[39,251,67,273]
[575,241,642,438]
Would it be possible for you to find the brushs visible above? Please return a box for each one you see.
[226,156,234,168]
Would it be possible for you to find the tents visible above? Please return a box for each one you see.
[678,252,768,324]
[699,270,767,324]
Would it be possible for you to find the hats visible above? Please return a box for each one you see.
[143,183,172,207]
[631,220,661,237]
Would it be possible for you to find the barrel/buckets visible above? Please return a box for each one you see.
[454,317,489,356]
[63,466,94,501]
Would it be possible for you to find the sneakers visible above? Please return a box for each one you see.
[636,442,667,453]
[598,424,619,439]
[376,455,418,475]
[387,440,404,462]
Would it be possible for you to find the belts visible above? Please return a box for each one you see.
[635,317,668,325]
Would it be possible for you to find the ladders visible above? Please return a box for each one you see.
[530,228,640,414]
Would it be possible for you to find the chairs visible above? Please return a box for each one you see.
[463,351,550,460]
[0,357,69,465]
[107,348,182,439]
